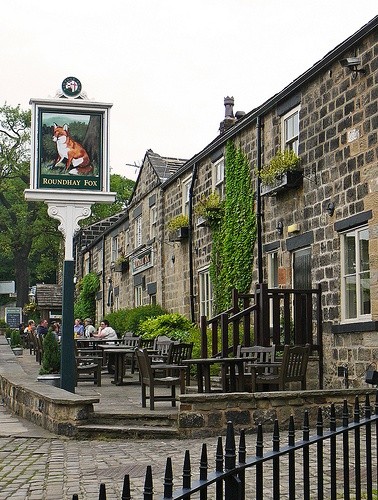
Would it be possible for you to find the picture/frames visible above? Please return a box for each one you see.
[22,97,117,204]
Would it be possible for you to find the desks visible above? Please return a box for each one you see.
[103,348,159,386]
[98,343,134,378]
[77,339,125,345]
[182,357,258,393]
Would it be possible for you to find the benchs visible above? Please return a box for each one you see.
[246,343,311,391]
[226,344,277,392]
[73,351,101,387]
[118,335,142,372]
[148,334,182,364]
[74,341,102,370]
[19,329,43,365]
[121,332,133,344]
[134,347,186,410]
[155,342,194,387]
[131,348,148,375]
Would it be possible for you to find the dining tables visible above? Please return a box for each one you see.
[74,336,85,340]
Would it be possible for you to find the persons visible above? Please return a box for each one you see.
[95,319,119,344]
[19,315,94,344]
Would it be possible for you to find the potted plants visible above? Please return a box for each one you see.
[193,191,223,227]
[168,214,189,241]
[114,250,129,272]
[254,149,303,198]
